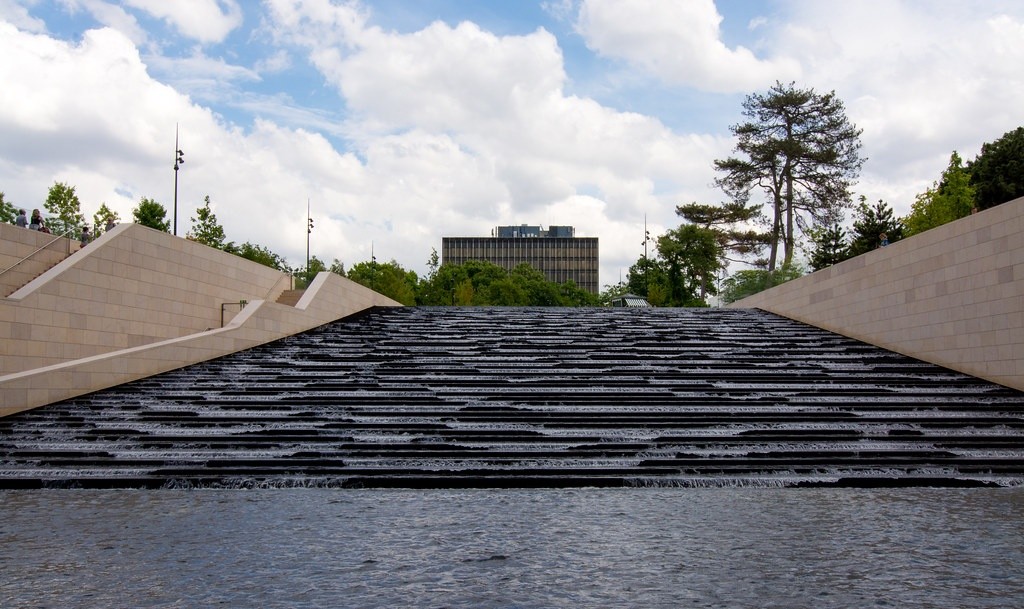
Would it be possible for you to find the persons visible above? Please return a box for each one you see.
[15,208,29,228]
[878,233,889,248]
[970,207,978,214]
[29,208,44,231]
[79,227,93,249]
[105,217,117,231]
